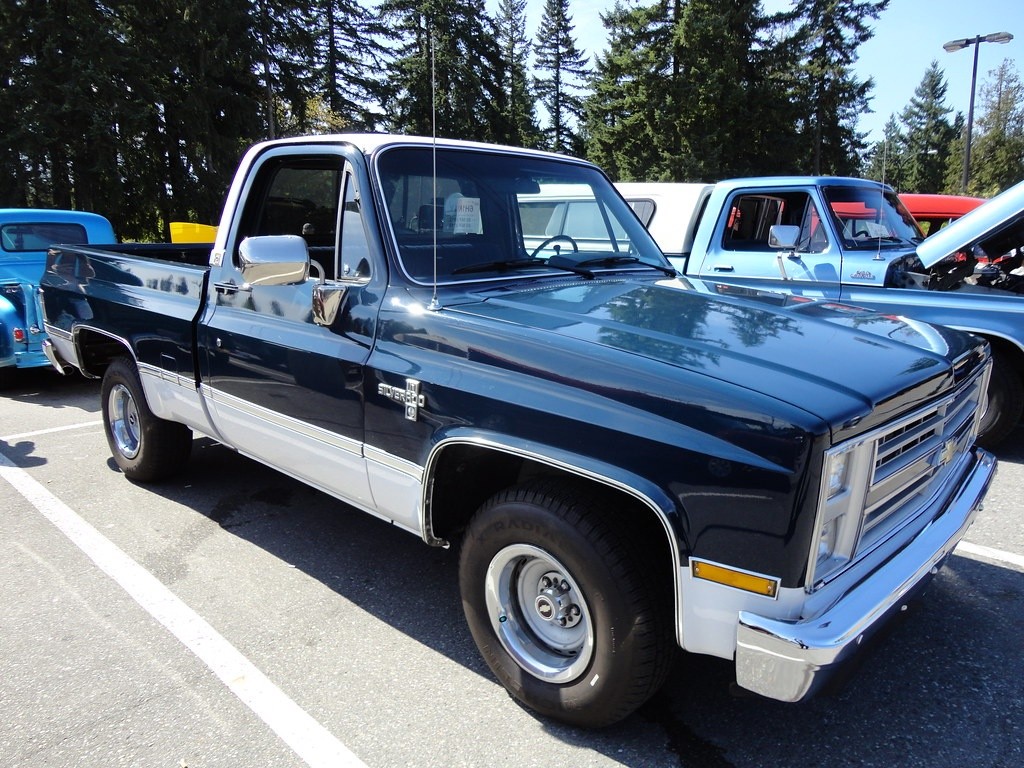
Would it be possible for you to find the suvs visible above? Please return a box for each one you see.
[516,173,1022,447]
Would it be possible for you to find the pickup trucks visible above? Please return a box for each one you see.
[39,134,998,730]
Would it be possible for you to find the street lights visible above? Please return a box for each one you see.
[942,32,1014,189]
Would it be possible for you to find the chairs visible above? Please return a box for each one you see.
[406,205,450,249]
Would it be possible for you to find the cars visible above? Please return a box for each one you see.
[725,194,1013,268]
[1,208,119,390]
